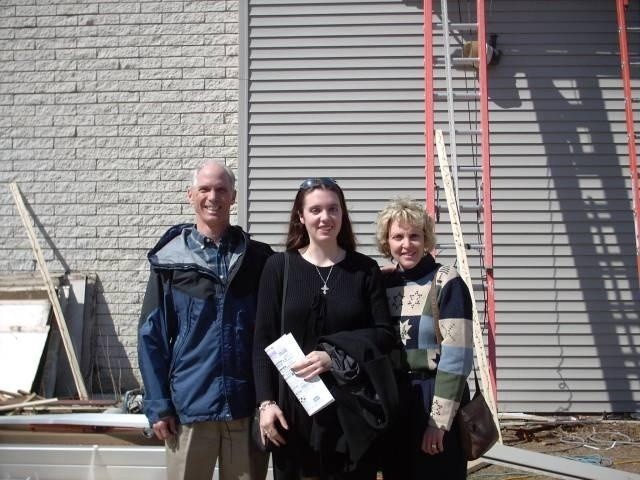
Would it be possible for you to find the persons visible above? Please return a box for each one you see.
[254,178,410,480]
[373,197,475,480]
[136,161,272,479]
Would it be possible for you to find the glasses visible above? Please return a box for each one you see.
[299,176,337,190]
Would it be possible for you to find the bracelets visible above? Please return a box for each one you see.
[257,399,279,410]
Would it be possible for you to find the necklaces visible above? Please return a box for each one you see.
[305,245,341,295]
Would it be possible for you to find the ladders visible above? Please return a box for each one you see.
[423,0,500,417]
[616,0,640,280]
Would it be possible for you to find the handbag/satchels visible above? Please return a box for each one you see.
[432,265,500,464]
[250,251,291,454]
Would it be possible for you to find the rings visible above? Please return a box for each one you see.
[431,444,438,449]
